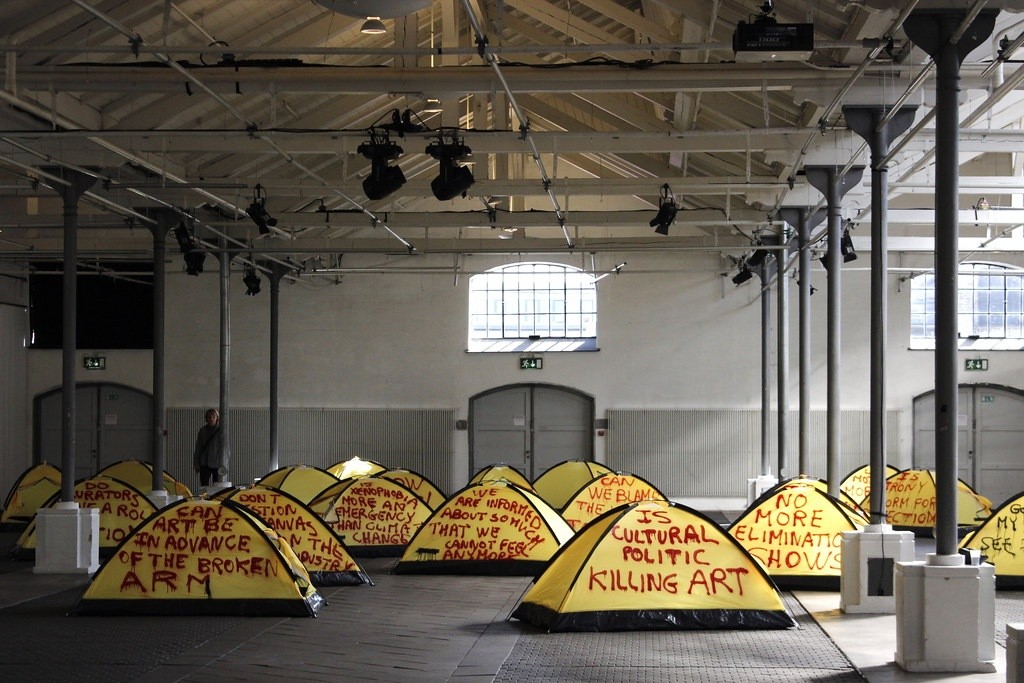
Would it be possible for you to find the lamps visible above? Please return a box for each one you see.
[360,16,387,35]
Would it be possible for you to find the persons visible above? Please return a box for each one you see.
[191,409,224,487]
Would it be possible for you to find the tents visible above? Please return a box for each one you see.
[92,458,195,501]
[506,501,805,632]
[726,479,872,592]
[837,460,904,508]
[957,490,1024,591]
[199,482,375,590]
[856,466,997,538]
[8,474,161,560]
[466,463,538,501]
[257,457,448,558]
[747,474,871,523]
[557,468,668,533]
[0,458,63,528]
[532,457,616,509]
[64,492,332,620]
[389,475,578,576]
